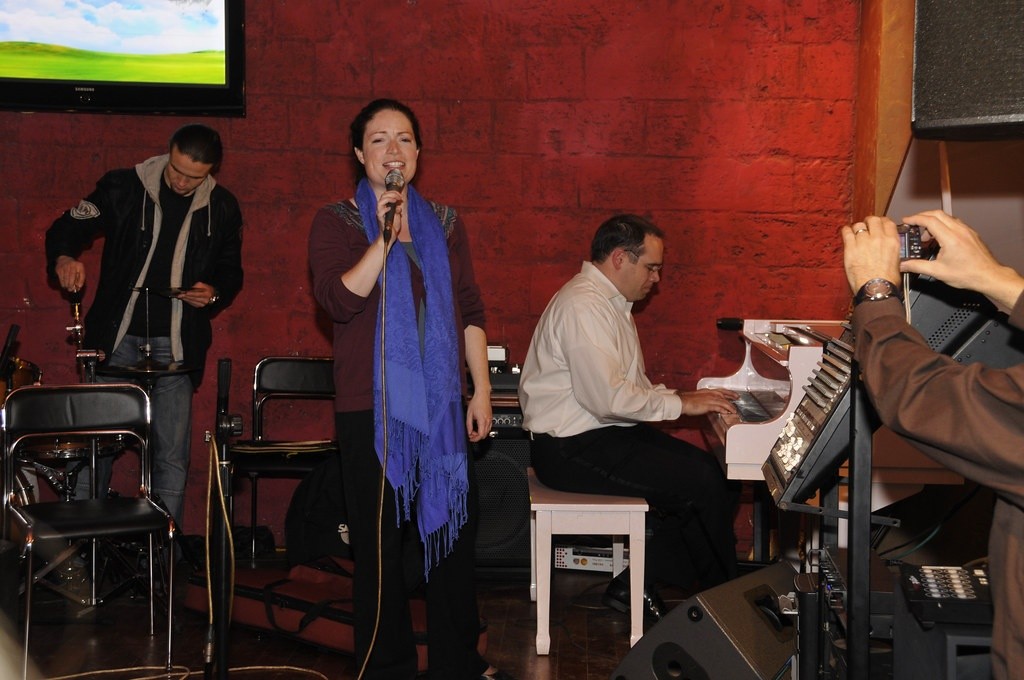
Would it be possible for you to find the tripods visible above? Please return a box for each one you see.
[18,362,174,622]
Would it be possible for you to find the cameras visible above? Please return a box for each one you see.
[895,222,921,261]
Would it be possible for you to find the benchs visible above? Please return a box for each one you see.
[526,466,649,655]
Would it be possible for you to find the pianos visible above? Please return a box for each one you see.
[693,315,968,569]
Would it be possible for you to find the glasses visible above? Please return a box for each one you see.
[619,247,663,273]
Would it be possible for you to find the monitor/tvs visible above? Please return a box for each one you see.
[0,0,247,118]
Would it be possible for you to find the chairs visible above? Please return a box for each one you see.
[0,383,176,680]
[247,356,339,569]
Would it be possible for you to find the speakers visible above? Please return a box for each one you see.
[608,558,800,680]
[469,412,538,568]
[908,0,1024,140]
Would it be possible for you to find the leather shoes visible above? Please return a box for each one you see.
[600,577,663,623]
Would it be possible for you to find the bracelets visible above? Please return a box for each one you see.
[675,390,685,398]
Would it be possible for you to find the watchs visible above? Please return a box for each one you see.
[852,278,901,308]
[208,287,220,306]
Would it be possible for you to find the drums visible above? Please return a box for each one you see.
[20,435,125,461]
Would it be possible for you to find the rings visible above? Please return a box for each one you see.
[855,228,869,235]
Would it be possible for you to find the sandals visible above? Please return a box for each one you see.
[478,665,514,680]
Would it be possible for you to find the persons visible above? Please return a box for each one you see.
[517,212,742,631]
[308,97,518,680]
[45,122,245,614]
[841,208,1024,680]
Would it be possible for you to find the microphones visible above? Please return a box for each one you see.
[204,623,215,680]
[382,168,405,243]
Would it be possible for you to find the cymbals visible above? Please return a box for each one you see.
[96,362,202,383]
[129,283,208,301]
